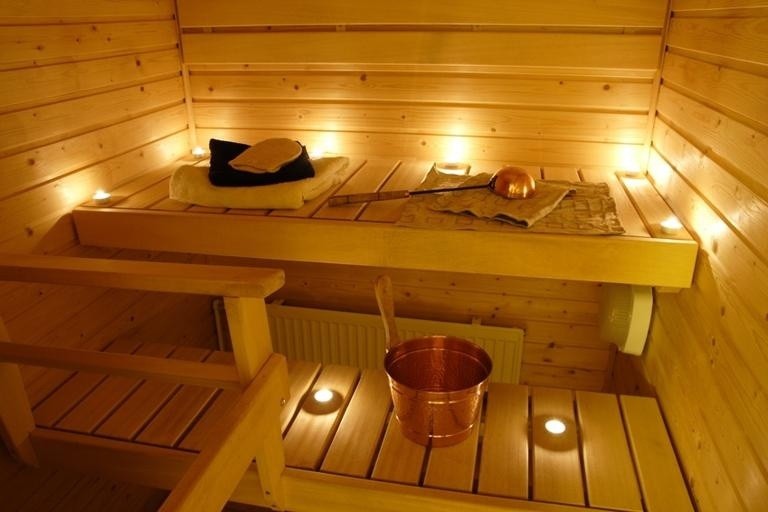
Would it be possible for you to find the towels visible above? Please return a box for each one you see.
[168,137,351,210]
[395,161,626,237]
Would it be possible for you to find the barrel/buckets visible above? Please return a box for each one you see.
[384,334,494,448]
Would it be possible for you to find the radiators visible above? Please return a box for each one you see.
[264,300,525,386]
